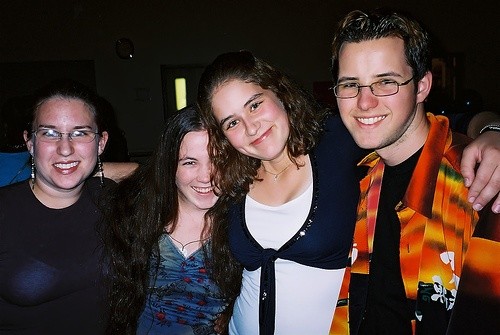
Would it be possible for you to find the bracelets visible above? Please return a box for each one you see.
[479,121,500,133]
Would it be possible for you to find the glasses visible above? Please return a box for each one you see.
[29,129,102,143]
[334,75,418,99]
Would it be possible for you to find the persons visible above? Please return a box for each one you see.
[195,49,500,335]
[328,10,500,335]
[0,152,139,187]
[0,82,118,335]
[98,105,245,335]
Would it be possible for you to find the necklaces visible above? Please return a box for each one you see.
[169,235,210,257]
[264,163,292,182]
